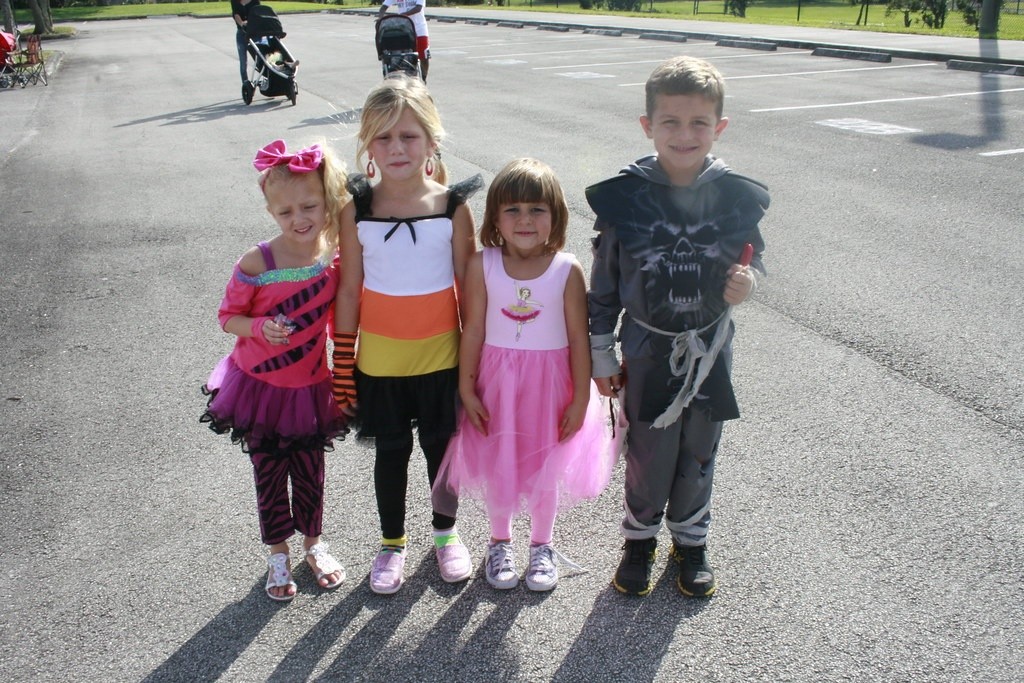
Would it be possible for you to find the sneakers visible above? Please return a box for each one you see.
[525,541,558,591]
[433,529,474,583]
[669,537,715,598]
[371,544,408,597]
[611,536,660,597]
[483,538,518,589]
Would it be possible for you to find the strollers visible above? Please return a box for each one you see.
[0,28,26,90]
[235,6,302,106]
[373,13,421,80]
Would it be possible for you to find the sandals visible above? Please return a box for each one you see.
[266,551,297,601]
[301,541,346,588]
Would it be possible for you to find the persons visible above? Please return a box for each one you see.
[229,0,263,97]
[580,55,772,596]
[331,78,476,592]
[377,0,432,86]
[263,46,299,71]
[199,140,355,599]
[427,159,631,596]
